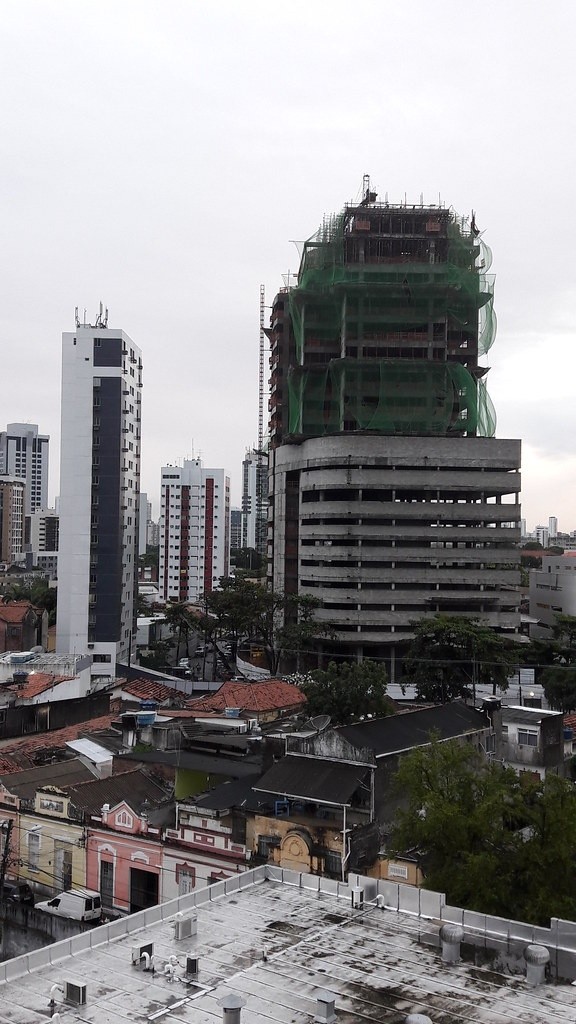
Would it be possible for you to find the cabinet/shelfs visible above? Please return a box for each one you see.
[275,801,290,818]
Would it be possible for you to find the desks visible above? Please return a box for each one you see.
[319,808,344,820]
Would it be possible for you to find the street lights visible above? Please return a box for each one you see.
[1,824,42,886]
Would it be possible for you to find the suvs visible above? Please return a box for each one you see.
[3,880,36,908]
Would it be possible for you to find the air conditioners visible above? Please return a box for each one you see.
[238,724,248,734]
[62,979,91,1004]
[131,941,160,965]
[280,709,287,717]
[248,718,259,731]
[172,914,202,942]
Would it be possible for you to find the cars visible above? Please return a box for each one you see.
[178,627,237,677]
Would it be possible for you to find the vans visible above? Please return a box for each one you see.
[36,889,103,923]
